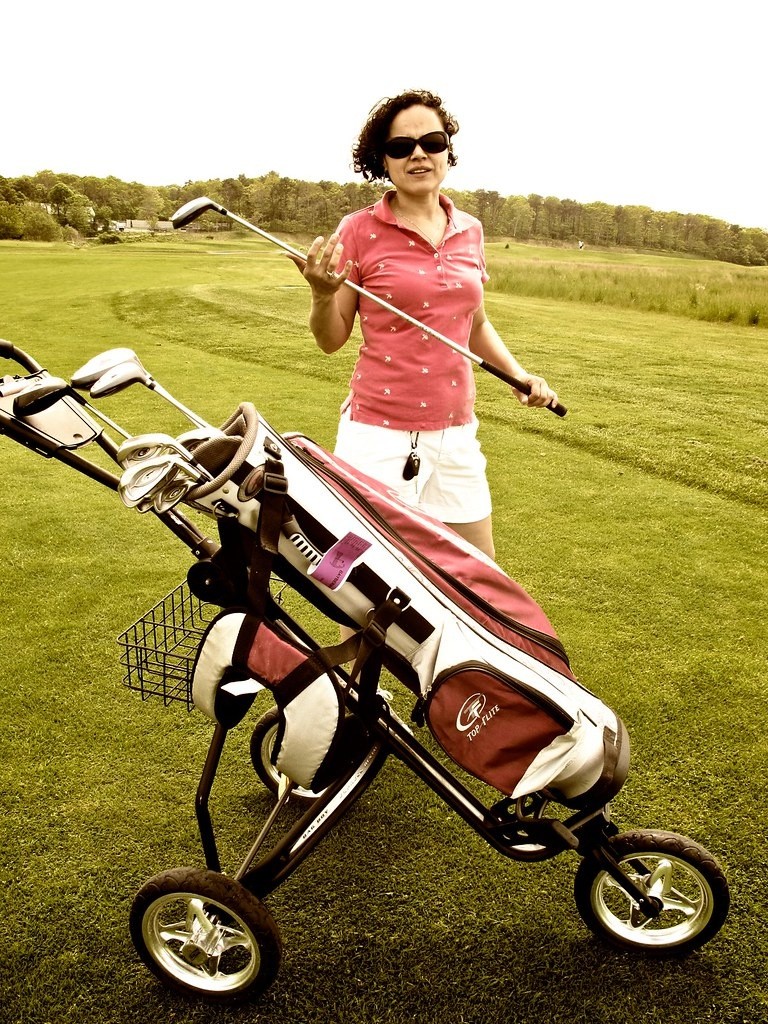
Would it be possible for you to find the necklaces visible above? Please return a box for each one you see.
[391,204,447,248]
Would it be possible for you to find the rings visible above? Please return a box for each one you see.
[325,269,335,278]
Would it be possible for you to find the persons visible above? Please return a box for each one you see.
[286,88,557,742]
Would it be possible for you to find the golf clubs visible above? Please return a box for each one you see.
[168,195,569,419]
[11,346,227,519]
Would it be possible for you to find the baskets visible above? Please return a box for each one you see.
[117,581,224,712]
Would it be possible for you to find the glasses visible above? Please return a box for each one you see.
[379,130,451,159]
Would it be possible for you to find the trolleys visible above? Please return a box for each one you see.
[0,338,731,1003]
